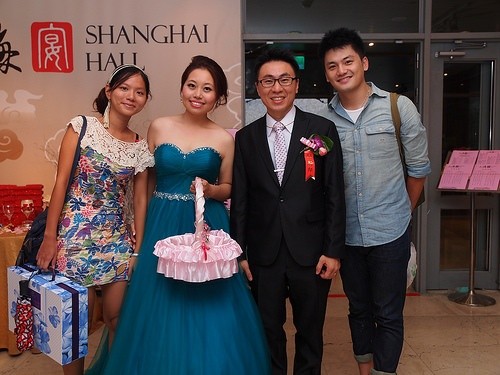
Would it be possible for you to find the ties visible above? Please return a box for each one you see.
[271,121,287,186]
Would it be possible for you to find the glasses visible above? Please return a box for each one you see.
[257,77,298,88]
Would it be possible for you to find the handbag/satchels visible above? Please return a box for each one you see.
[15,207,53,267]
[405,243,417,288]
[389,92,426,208]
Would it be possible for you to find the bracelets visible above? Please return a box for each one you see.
[131,253,138,256]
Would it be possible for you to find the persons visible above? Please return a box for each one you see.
[84,55,267,375]
[36,63,155,375]
[315,27,431,375]
[229,51,346,375]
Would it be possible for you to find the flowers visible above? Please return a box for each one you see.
[300,134,335,156]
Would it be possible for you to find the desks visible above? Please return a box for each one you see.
[0,230,104,355]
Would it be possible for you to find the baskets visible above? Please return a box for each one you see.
[155,177,242,281]
